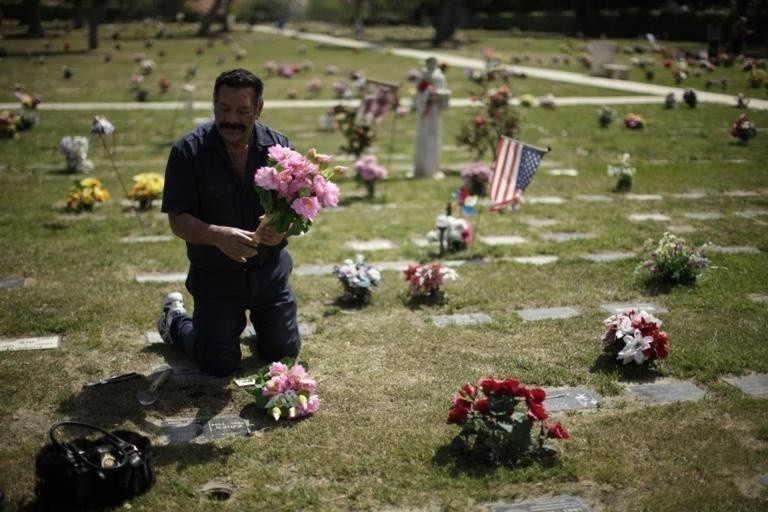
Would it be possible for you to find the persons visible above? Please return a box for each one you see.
[706,16,755,60]
[412,57,451,179]
[156,69,302,377]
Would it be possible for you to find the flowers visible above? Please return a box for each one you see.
[249,142,346,242]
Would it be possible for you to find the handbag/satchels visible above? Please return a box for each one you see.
[34,421,156,512]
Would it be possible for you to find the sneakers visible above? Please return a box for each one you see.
[157,292,186,344]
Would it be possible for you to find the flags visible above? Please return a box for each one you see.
[485,136,545,215]
